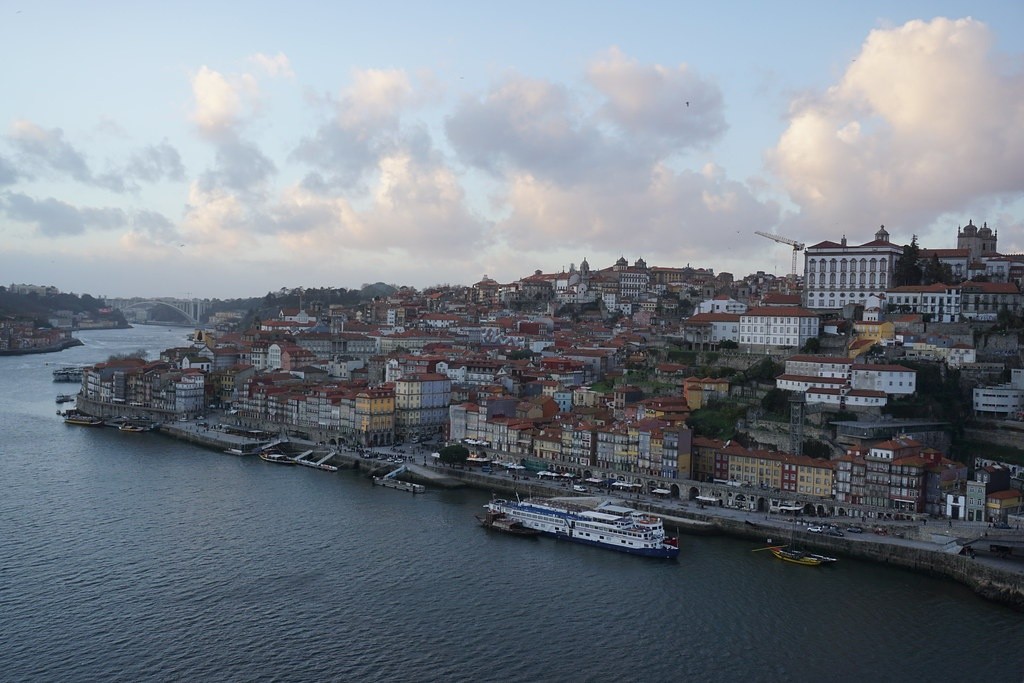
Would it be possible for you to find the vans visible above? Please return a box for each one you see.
[574,484,587,492]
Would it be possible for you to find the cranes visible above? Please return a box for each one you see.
[754,231,805,295]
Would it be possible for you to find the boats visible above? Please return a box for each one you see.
[482,491,680,560]
[475,510,542,538]
[769,547,821,566]
[65,419,102,426]
[791,550,836,563]
[118,426,143,432]
[56,392,64,403]
[258,453,296,464]
[52,367,83,383]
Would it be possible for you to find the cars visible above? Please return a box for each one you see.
[825,529,844,538]
[807,526,823,534]
[102,412,150,421]
[482,466,491,472]
[410,436,420,444]
[847,527,863,534]
[361,453,403,464]
